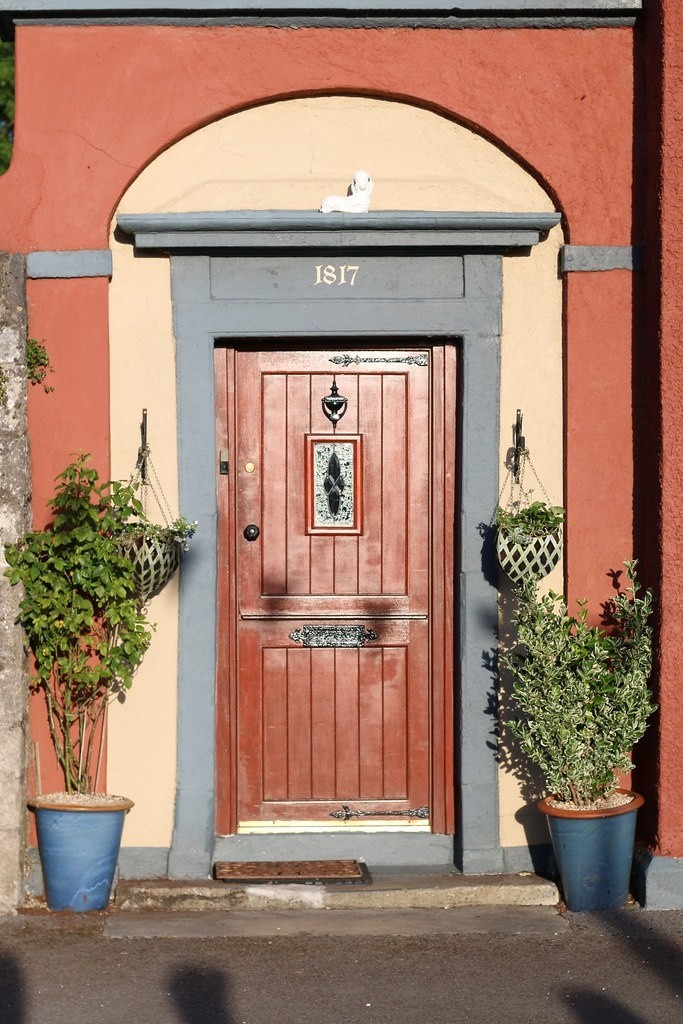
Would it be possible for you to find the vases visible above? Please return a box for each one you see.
[115,534,179,597]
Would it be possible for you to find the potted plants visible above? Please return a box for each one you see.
[491,498,566,583]
[498,560,661,912]
[3,454,149,918]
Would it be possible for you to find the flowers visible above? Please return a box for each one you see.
[107,514,201,551]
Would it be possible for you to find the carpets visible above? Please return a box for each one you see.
[214,860,375,888]
